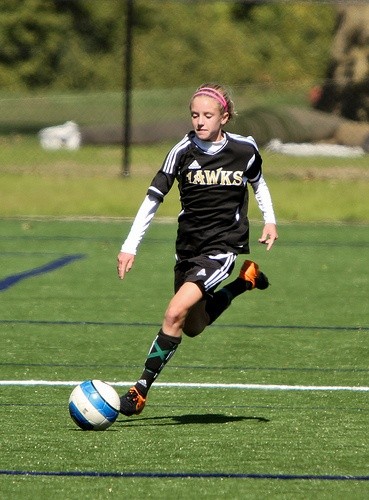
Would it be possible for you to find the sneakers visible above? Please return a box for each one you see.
[240,259,272,291]
[118,386,147,418]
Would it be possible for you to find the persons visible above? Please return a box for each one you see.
[117,84,279,422]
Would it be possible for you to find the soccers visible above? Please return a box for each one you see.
[67,379,120,431]
